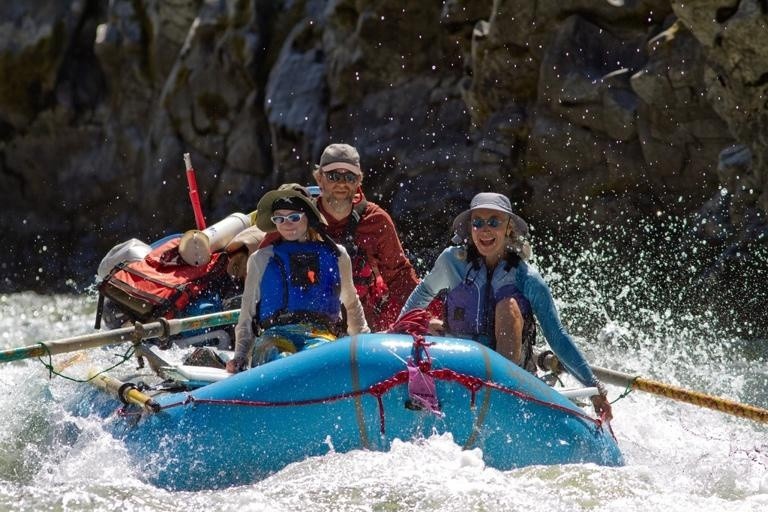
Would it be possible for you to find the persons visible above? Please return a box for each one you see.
[396,192,613,422]
[226,184,371,372]
[258,144,445,335]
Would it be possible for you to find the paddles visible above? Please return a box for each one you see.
[49,352,161,414]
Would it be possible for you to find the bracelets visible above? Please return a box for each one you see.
[590,382,607,399]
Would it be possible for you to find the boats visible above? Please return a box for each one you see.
[56,331,632,495]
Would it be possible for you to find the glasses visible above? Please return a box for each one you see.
[471,215,503,228]
[270,212,306,226]
[321,172,361,182]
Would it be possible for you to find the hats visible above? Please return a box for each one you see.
[319,143,363,177]
[254,182,329,234]
[452,192,529,241]
